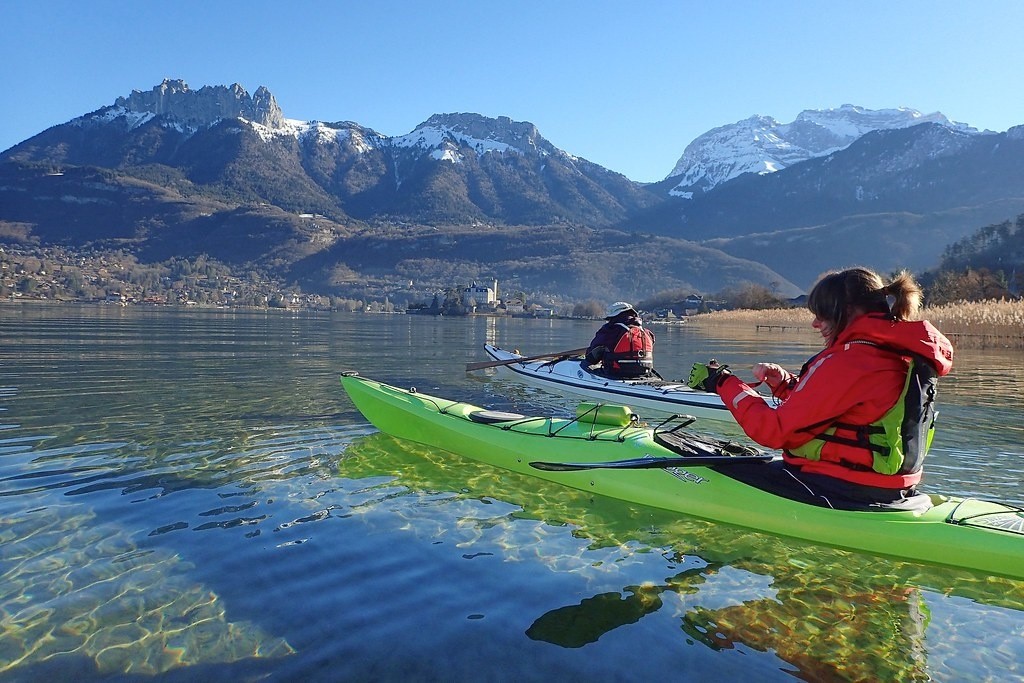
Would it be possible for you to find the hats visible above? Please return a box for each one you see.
[602,302,638,321]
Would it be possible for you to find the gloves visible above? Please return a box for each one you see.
[686,359,728,393]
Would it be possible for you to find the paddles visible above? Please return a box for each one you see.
[464,345,589,373]
[528,454,774,474]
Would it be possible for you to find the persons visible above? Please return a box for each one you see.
[585,300,655,383]
[687,266,954,512]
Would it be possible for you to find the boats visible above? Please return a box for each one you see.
[335,365,1024,578]
[483,340,784,439]
[339,429,1023,614]
